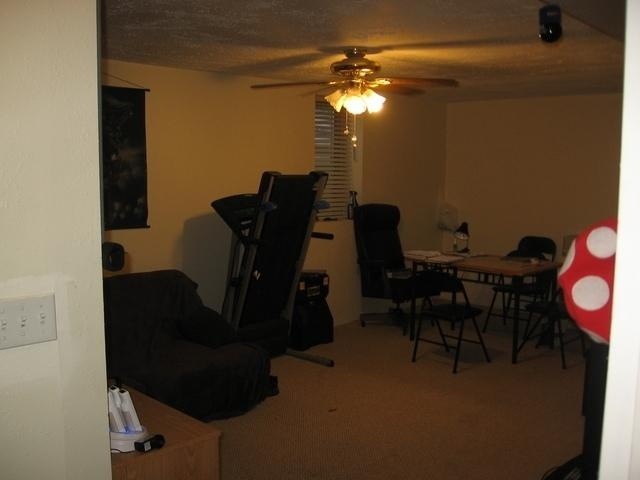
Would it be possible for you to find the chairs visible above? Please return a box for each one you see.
[352,202,442,340]
[405,235,586,376]
[103,267,284,423]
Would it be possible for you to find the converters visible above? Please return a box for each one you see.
[134,434,165,453]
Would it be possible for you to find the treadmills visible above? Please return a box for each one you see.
[210,170,334,372]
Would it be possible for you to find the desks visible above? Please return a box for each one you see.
[106,379,226,479]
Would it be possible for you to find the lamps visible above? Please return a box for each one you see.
[322,80,385,150]
[453,222,471,252]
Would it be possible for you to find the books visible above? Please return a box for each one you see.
[427,254,466,262]
[405,249,439,261]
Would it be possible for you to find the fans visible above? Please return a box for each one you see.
[251,45,460,102]
